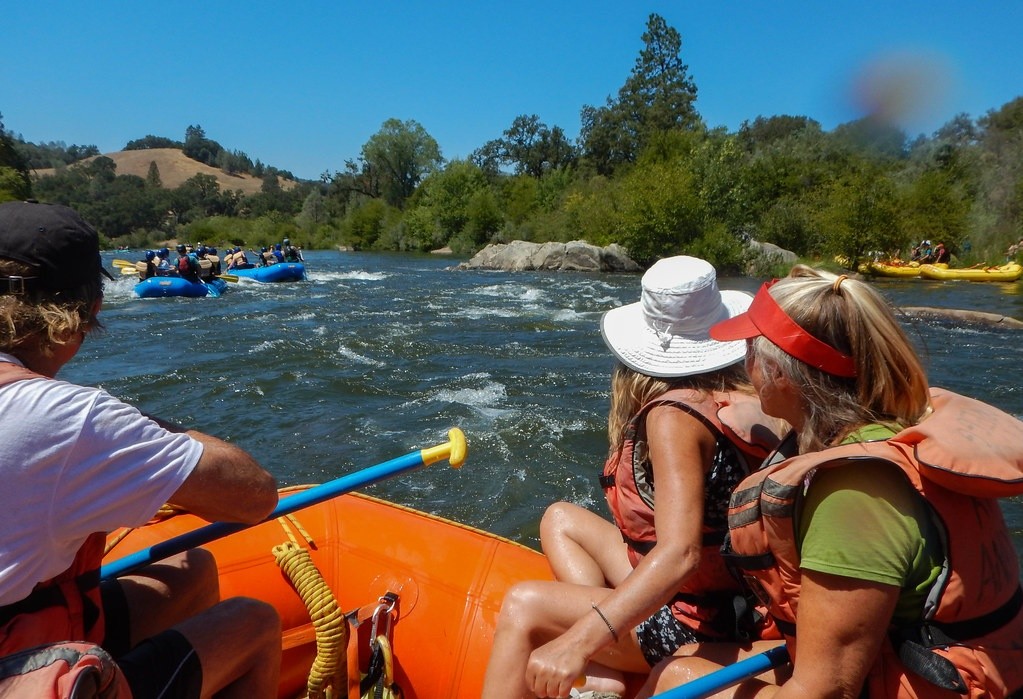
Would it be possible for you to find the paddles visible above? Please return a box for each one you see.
[102,426,468,579]
[122,267,141,276]
[653,640,787,696]
[112,258,240,283]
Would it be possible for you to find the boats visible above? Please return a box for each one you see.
[133,278,227,297]
[225,263,305,282]
[835,253,1021,281]
[100,483,653,699]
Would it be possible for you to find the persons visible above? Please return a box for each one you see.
[919,241,936,263]
[911,244,921,261]
[633,263,1023,699]
[932,240,951,263]
[481,253,793,699]
[959,235,971,255]
[280,238,300,263]
[137,244,278,286]
[1017,238,1023,253]
[1,202,281,699]
[1004,242,1019,262]
[270,244,289,263]
[891,256,905,266]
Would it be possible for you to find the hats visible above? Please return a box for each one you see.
[600,256,762,378]
[0,198,115,302]
[710,277,860,377]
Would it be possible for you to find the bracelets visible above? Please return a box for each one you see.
[593,604,619,643]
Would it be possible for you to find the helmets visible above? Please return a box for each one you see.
[145,240,290,262]
